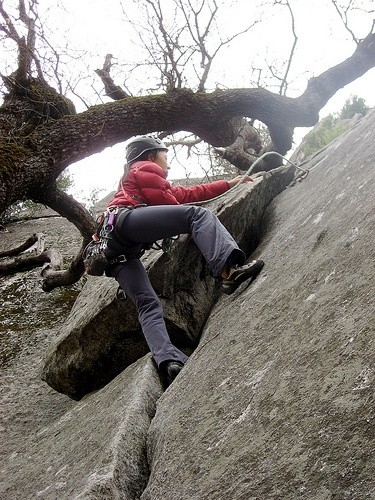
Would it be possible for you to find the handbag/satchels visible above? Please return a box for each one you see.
[82,224,114,277]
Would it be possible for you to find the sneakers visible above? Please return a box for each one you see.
[167,361,184,380]
[220,259,264,295]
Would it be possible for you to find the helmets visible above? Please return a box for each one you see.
[125,135,169,164]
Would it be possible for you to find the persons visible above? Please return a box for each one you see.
[105,135,265,381]
